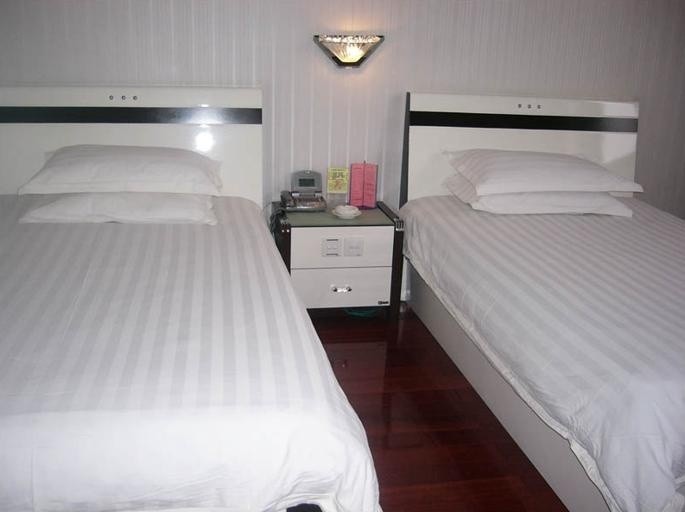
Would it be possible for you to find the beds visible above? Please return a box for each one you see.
[0,80,385,512]
[400,90,685,511]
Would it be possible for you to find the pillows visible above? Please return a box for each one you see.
[440,148,645,218]
[16,144,221,228]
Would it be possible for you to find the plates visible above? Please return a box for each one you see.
[331,209,362,220]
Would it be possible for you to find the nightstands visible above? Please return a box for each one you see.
[271,200,404,335]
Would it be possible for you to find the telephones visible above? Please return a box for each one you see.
[280,171,327,212]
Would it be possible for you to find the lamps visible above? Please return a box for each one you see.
[312,34,384,66]
